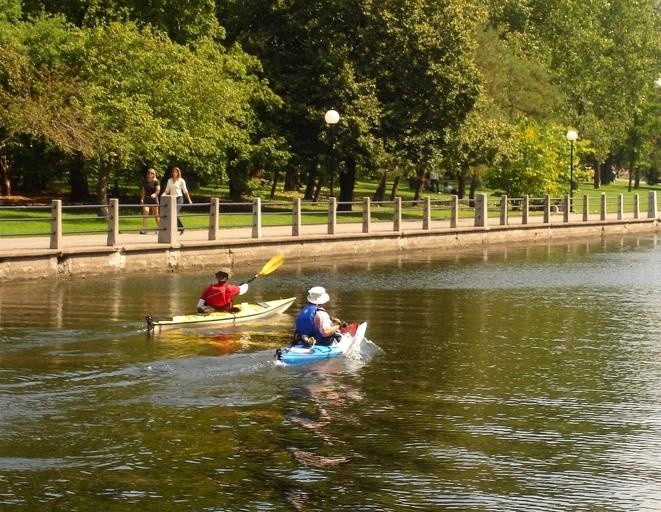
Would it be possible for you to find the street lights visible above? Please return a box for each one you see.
[324,110,340,196]
[567,130,578,212]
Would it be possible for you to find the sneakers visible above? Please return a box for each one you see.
[138,227,185,235]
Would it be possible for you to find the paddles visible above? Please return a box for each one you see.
[248,253,285,284]
[338,322,367,344]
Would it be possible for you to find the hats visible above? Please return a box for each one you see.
[306,286,330,305]
[215,267,231,279]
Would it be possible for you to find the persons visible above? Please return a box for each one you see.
[140,167,192,235]
[296,287,348,346]
[424,171,459,195]
[197,268,249,310]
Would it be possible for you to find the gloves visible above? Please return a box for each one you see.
[339,321,348,331]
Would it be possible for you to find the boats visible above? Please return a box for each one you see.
[273,321,368,366]
[146,296,296,334]
